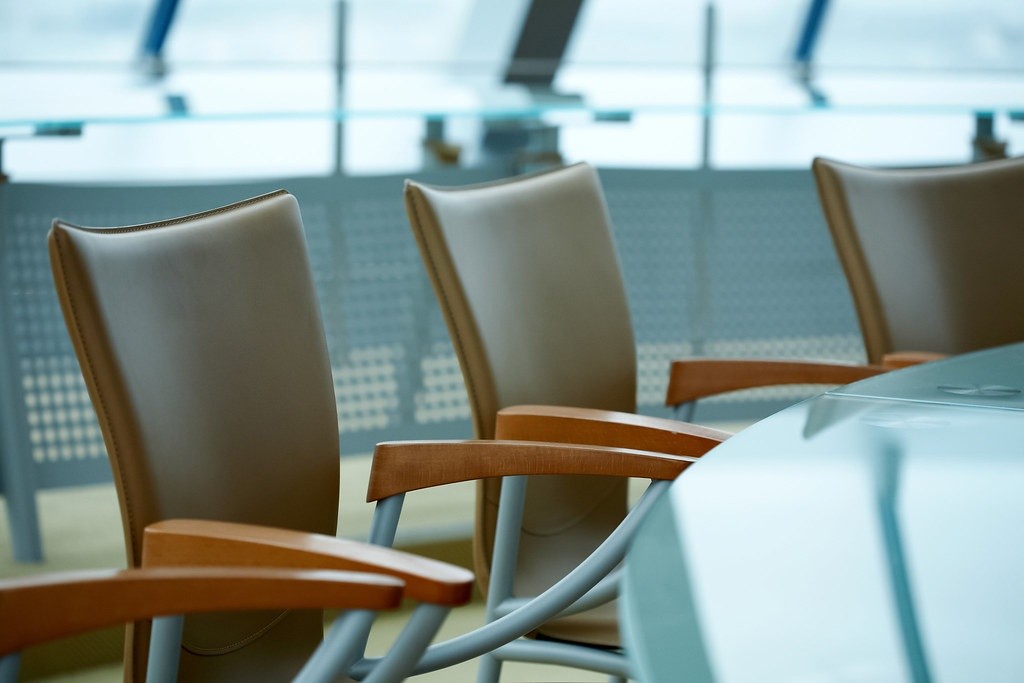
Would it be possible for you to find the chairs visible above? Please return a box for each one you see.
[813,152,1024,367]
[403,159,896,683]
[47,188,695,683]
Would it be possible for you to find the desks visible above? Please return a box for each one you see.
[620,342,1024,683]
